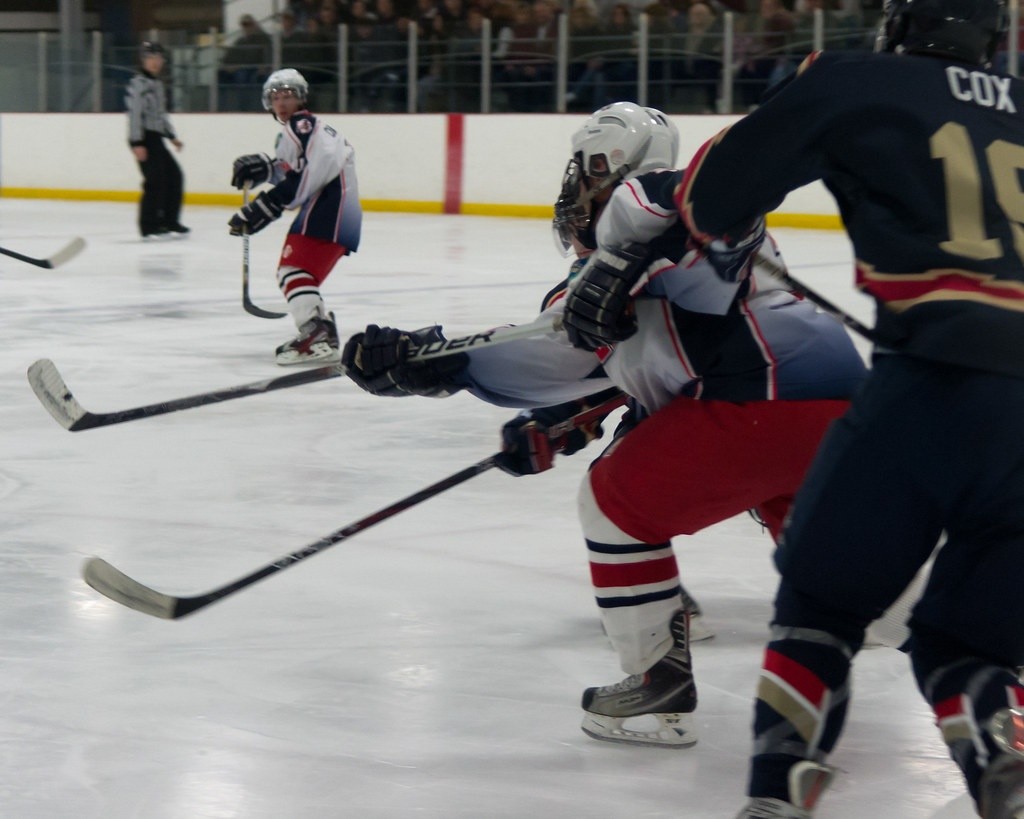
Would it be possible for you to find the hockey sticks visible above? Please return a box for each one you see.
[240,179,288,319]
[23,317,566,435]
[0,236,86,270]
[77,393,627,622]
[753,252,875,343]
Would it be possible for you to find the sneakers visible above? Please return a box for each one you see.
[271,311,342,367]
[731,759,840,819]
[140,221,191,243]
[577,609,700,749]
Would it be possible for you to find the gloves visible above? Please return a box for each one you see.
[341,323,473,400]
[560,243,650,353]
[492,395,604,478]
[227,189,285,237]
[687,214,768,286]
[230,151,274,191]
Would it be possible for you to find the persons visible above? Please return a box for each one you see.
[671,0,1024,819]
[226,68,361,367]
[340,101,934,748]
[124,41,189,239]
[276,0,1024,111]
[217,13,273,85]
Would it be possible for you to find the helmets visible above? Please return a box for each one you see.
[261,68,309,112]
[138,40,166,56]
[554,100,679,259]
[872,0,1011,68]
